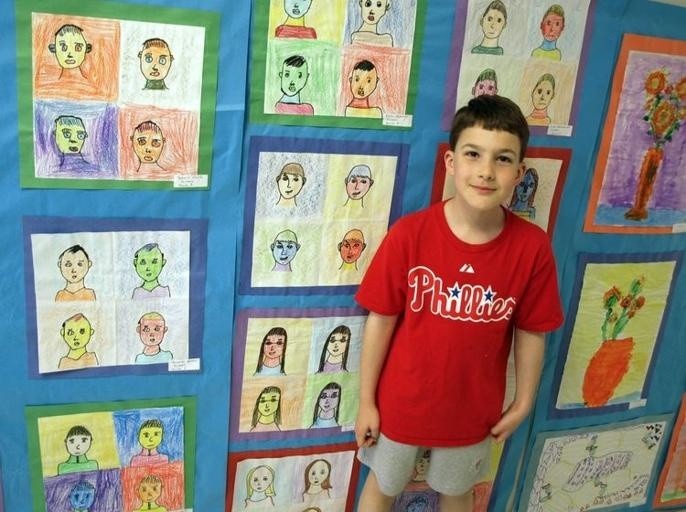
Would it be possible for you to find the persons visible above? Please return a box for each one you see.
[354,94,566,511]
